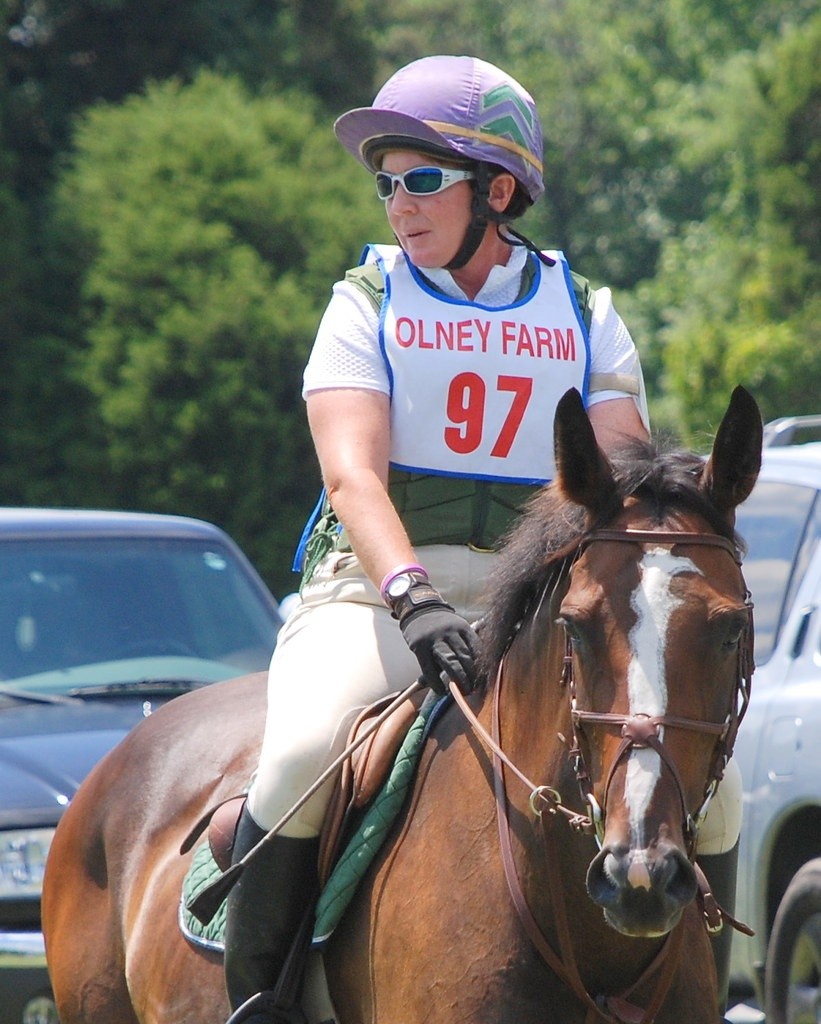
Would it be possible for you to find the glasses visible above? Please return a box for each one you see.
[374,165,475,200]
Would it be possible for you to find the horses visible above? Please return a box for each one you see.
[41,385,764,1023]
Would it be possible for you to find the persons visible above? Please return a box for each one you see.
[216,55,645,1024]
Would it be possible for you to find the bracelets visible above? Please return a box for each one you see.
[378,560,428,609]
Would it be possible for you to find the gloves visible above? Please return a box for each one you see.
[379,565,484,698]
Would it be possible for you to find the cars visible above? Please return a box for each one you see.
[0,506,299,1024]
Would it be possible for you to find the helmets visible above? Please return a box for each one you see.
[333,56,546,205]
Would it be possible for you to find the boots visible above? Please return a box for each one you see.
[220,804,308,1024]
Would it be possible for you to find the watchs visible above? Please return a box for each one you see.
[385,572,433,620]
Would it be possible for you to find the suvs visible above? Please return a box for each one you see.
[613,416,821,1024]
